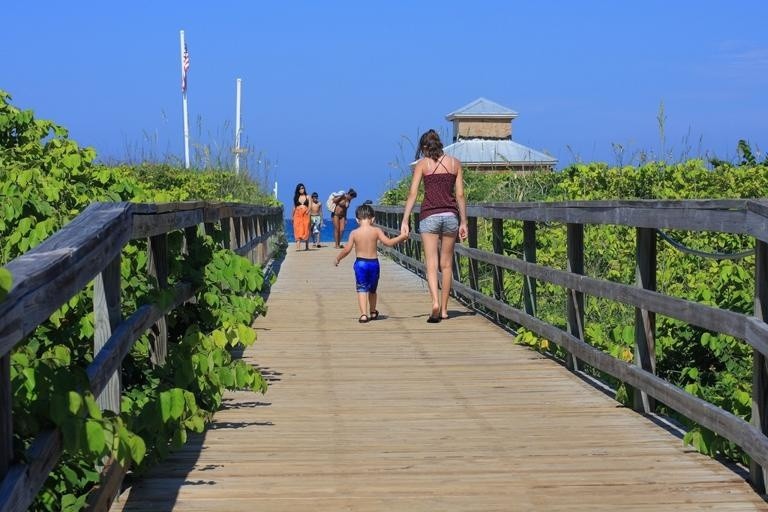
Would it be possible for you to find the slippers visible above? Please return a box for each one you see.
[426,314,439,323]
[439,312,449,320]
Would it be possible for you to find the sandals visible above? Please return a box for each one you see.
[358,314,368,323]
[371,310,379,320]
[295,241,344,253]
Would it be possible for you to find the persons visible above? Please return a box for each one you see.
[400,129,469,323]
[310,192,323,247]
[291,183,312,252]
[334,205,410,323]
[331,188,357,249]
